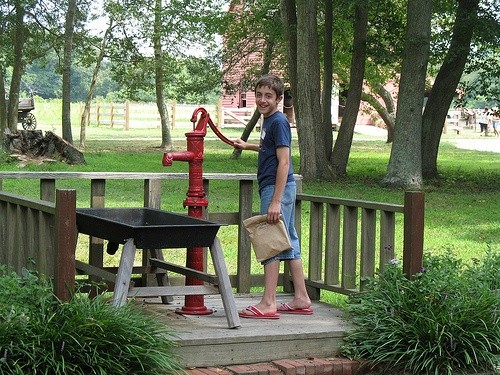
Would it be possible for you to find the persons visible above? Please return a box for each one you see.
[234,76,313,319]
[478,107,500,137]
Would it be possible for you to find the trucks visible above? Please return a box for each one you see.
[4,94,37,131]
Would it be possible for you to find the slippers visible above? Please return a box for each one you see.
[238,305,281,319]
[277,301,314,314]
